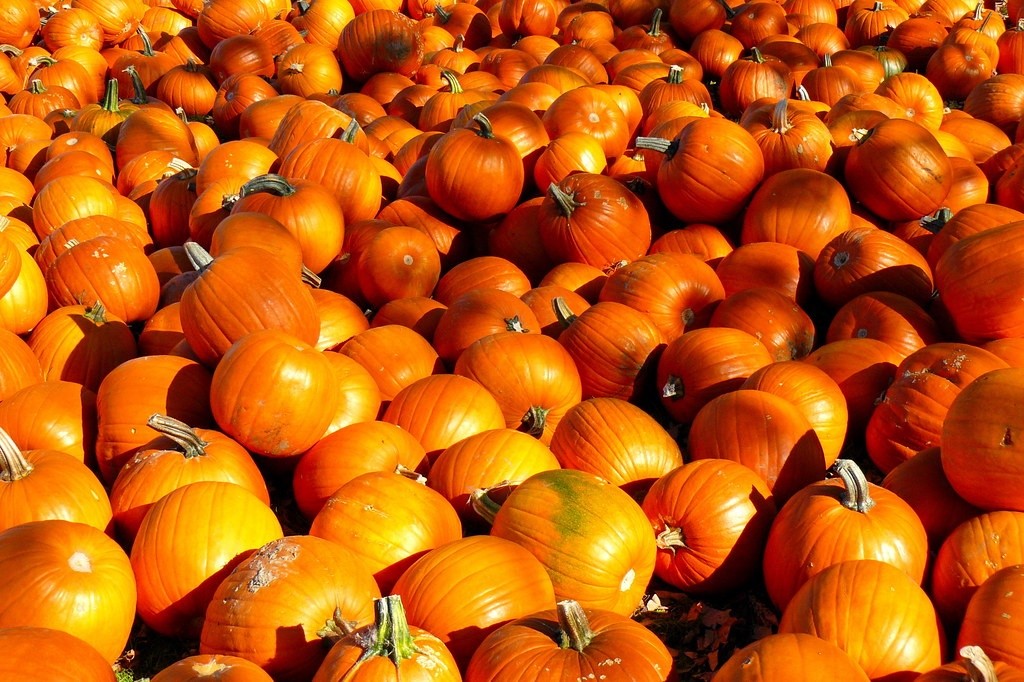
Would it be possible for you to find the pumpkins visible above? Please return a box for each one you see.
[0,0,1024,682]
